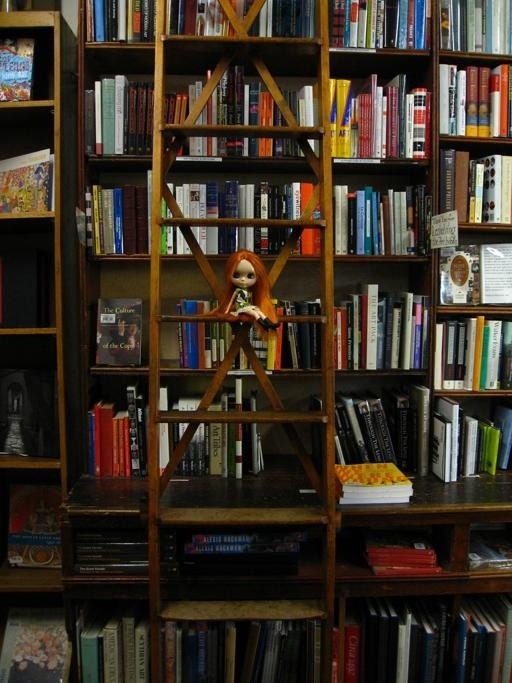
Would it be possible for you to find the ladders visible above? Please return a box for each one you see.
[150,1,335,682]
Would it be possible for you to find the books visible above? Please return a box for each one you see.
[329,1,433,51]
[330,73,430,157]
[177,296,324,369]
[162,63,322,157]
[95,298,142,367]
[85,170,152,256]
[334,463,414,504]
[160,181,323,256]
[333,283,428,370]
[84,75,154,157]
[164,1,316,39]
[432,60,510,390]
[158,378,261,477]
[2,508,512,683]
[86,380,150,479]
[312,384,512,483]
[86,1,156,42]
[332,184,431,256]
[439,62,511,140]
[436,1,511,54]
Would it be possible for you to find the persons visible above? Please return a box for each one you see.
[211,249,281,335]
